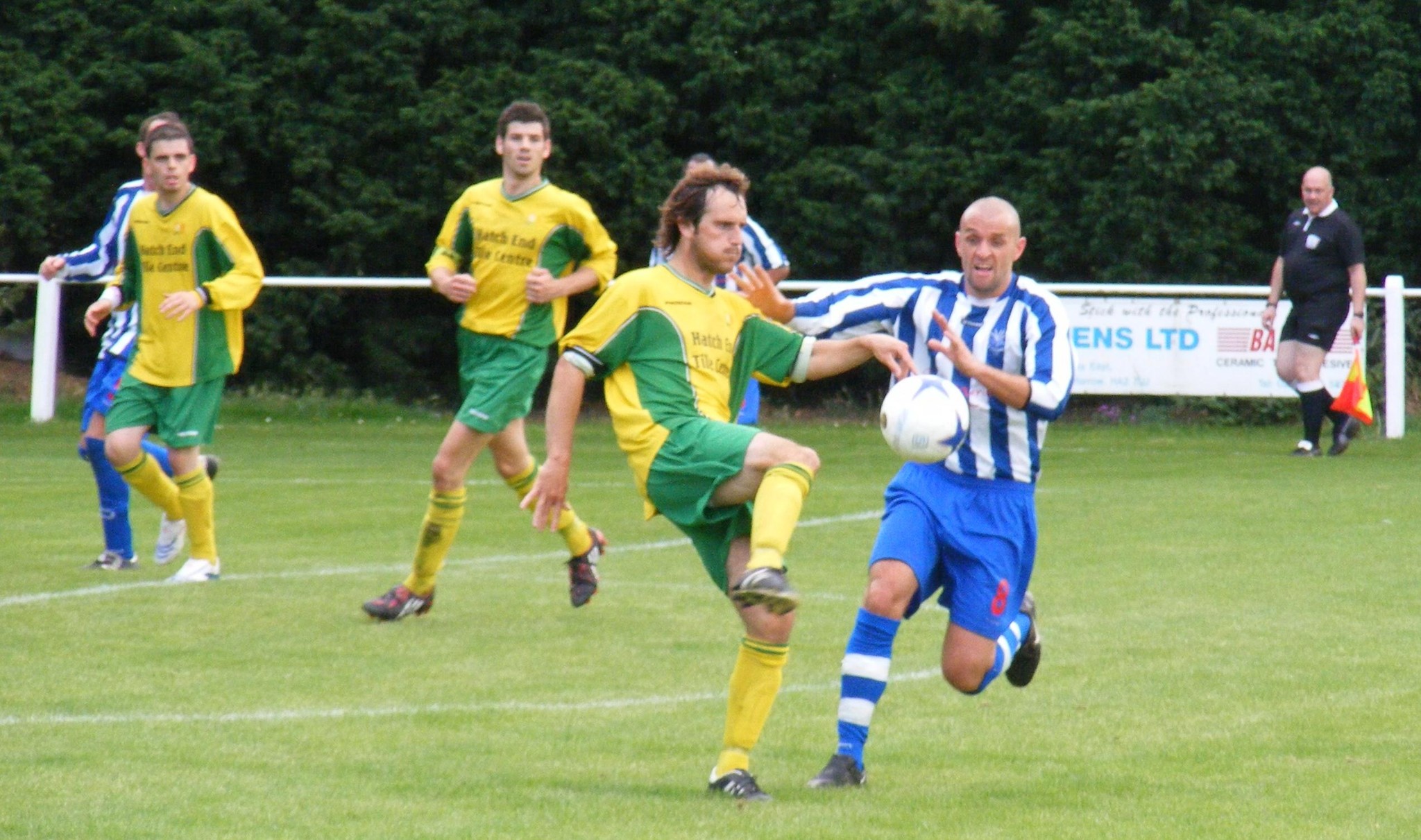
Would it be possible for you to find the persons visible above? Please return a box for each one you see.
[520,147,915,799]
[1261,165,1367,460]
[359,99,609,620]
[39,111,265,585]
[731,196,1075,791]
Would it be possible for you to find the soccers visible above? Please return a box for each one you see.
[881,374,970,465]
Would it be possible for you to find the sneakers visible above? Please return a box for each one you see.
[166,558,220,585]
[89,551,140,571]
[153,513,187,564]
[708,768,768,802]
[360,584,434,622]
[567,527,608,609]
[201,454,219,479]
[1292,440,1323,459]
[1007,593,1040,688]
[806,754,867,793]
[730,567,796,615]
[1328,417,1360,456]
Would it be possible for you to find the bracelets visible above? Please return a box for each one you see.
[1264,301,1280,308]
[192,284,212,306]
[1353,310,1364,319]
